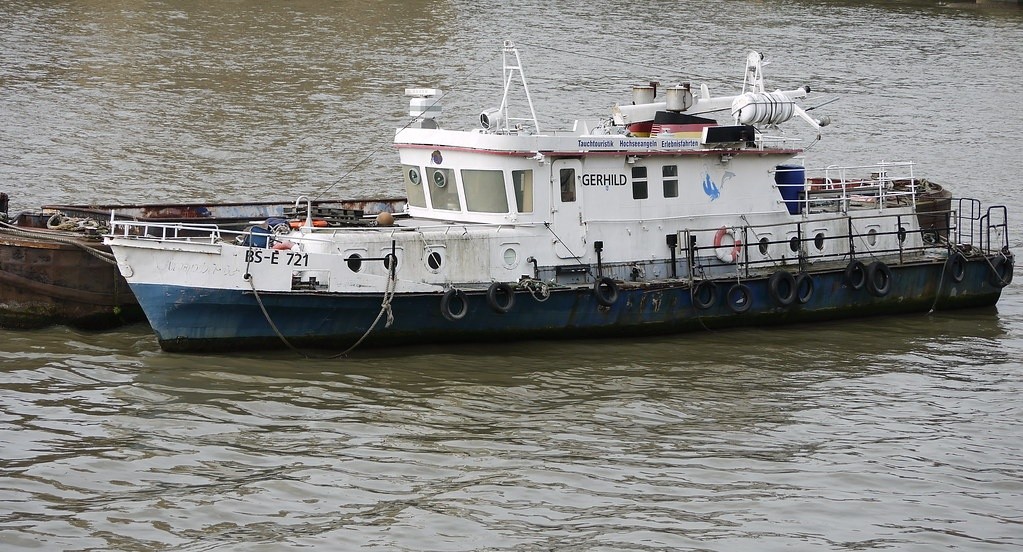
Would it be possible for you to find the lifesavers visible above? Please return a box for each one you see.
[793,273,814,303]
[945,253,966,284]
[486,280,516,314]
[843,260,868,291]
[689,279,719,310]
[593,278,619,308]
[713,224,744,265]
[725,284,755,314]
[283,219,331,229]
[865,262,893,296]
[439,288,471,322]
[986,258,1012,289]
[766,270,797,306]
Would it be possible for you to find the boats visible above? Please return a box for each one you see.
[96,35,1018,349]
[0,189,407,330]
